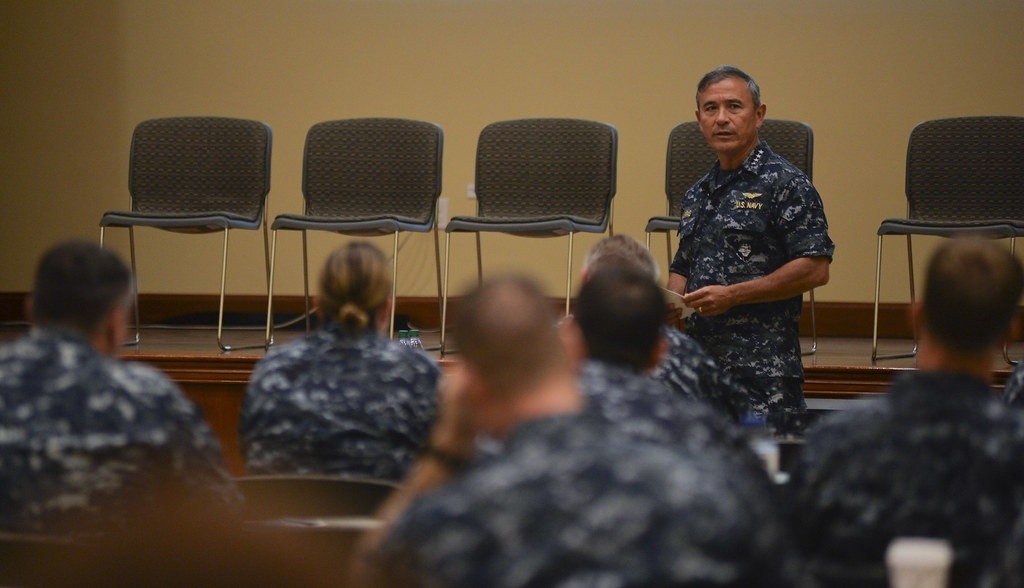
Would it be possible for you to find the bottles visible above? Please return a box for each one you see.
[398,330,411,348]
[409,330,423,349]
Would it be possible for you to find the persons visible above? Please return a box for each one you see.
[1,239,252,537]
[665,66,836,441]
[351,236,820,587]
[791,237,1023,588]
[241,241,444,484]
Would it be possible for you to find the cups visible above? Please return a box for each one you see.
[752,440,780,476]
[887,539,951,588]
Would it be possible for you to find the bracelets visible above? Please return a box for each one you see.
[421,447,467,473]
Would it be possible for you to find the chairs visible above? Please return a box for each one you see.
[870,114,1023,367]
[1,463,398,588]
[442,118,617,355]
[645,117,818,356]
[264,118,446,351]
[100,116,274,351]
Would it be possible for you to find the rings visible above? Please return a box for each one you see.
[698,307,703,313]
